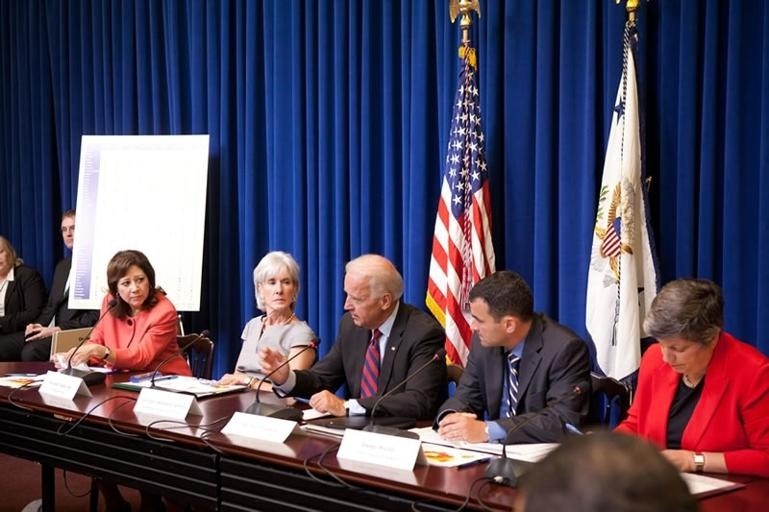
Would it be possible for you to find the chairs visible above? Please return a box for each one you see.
[582,372,632,430]
[84,328,218,512]
[443,364,487,424]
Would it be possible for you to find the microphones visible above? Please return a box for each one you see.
[363,348,447,439]
[246,338,321,422]
[149,330,210,399]
[60,300,118,386]
[483,381,590,491]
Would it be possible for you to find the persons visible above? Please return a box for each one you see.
[0,235,49,362]
[612,280,769,479]
[21,210,101,363]
[256,255,447,419]
[513,428,698,512]
[217,251,317,391]
[53,249,192,373]
[432,271,600,445]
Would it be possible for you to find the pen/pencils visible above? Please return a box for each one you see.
[150,375,178,382]
[293,397,310,404]
[3,373,37,377]
[565,423,583,435]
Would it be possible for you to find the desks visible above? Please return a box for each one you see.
[1,359,769,510]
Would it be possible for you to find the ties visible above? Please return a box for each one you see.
[360,328,383,398]
[505,352,522,418]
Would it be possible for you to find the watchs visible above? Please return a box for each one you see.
[343,399,349,417]
[695,451,704,472]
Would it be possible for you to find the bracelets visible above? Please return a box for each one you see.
[248,376,259,386]
[101,346,111,360]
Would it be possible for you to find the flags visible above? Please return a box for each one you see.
[575,21,668,408]
[424,47,495,383]
